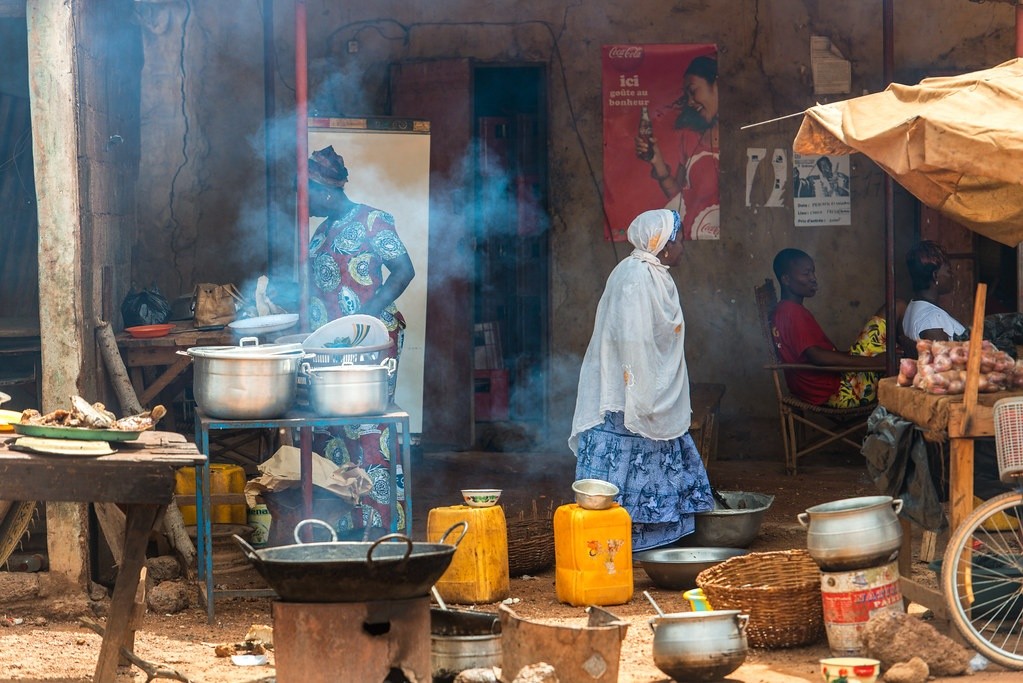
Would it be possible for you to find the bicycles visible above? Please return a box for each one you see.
[940,395,1023,672]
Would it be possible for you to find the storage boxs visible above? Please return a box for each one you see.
[472,321,510,421]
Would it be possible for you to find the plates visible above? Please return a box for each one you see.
[125,324,176,337]
[228,313,299,334]
[302,314,389,348]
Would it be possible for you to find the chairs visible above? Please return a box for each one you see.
[753,279,886,476]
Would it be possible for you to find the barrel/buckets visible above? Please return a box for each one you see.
[426,505,510,604]
[553,503,634,607]
[174,463,247,526]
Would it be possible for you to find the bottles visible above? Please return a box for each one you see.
[638,107,652,161]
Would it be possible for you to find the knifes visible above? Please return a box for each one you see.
[169,324,224,333]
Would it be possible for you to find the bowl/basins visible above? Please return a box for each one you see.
[819,657,880,683]
[632,547,749,590]
[572,479,620,509]
[674,490,775,548]
[461,489,503,507]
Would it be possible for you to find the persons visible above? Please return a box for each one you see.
[241,144,416,542]
[568,209,715,559]
[801,157,849,197]
[771,240,1023,531]
[630,55,719,240]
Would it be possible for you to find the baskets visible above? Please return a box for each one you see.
[695,548,825,650]
[992,396,1023,483]
[504,500,558,574]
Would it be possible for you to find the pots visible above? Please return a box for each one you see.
[648,610,749,683]
[175,336,316,419]
[302,357,396,417]
[430,605,502,635]
[797,495,903,572]
[232,519,468,602]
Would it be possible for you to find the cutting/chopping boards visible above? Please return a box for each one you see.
[115,320,235,347]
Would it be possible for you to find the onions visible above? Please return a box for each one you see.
[897,340,1023,395]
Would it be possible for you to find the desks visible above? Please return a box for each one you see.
[195,407,411,627]
[0,427,208,682]
[879,376,1023,650]
[690,382,725,477]
[116,319,264,433]
